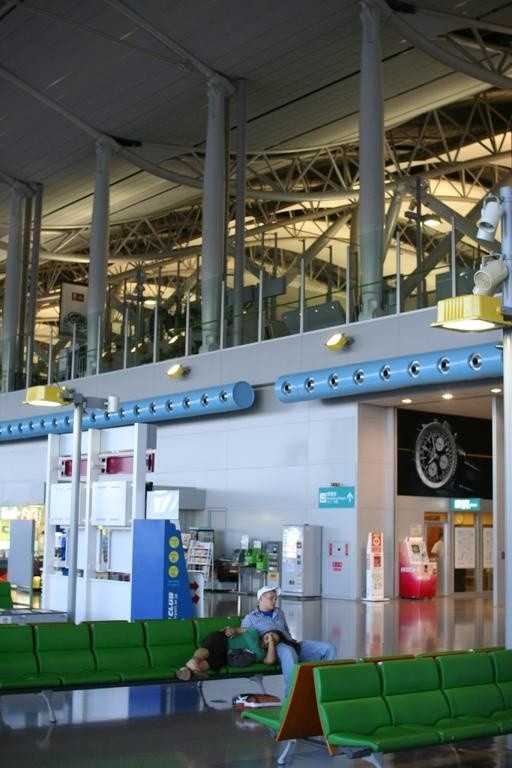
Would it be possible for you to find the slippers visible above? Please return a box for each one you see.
[176,668,208,681]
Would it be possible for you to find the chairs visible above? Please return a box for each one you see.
[312,650,512,768]
[0,616,284,723]
[241,646,502,768]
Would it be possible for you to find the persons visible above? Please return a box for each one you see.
[242,583,336,699]
[175,623,304,681]
[429,533,444,558]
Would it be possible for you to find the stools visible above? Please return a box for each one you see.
[257,570,268,587]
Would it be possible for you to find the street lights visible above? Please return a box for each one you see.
[22,383,120,622]
[426,185,511,653]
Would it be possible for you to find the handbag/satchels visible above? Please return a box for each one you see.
[227,649,255,666]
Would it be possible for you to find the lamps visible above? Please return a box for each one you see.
[422,214,441,227]
[476,193,503,243]
[168,364,190,378]
[472,253,510,297]
[325,333,353,350]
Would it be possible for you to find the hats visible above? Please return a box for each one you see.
[257,586,281,600]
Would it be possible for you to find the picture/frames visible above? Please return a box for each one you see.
[59,281,88,339]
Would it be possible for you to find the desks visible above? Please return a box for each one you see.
[223,563,256,595]
